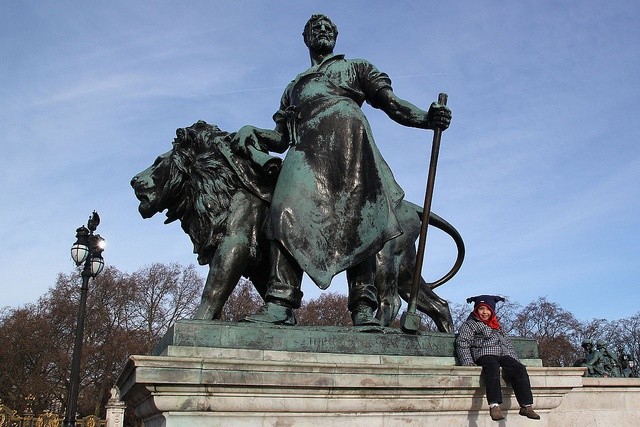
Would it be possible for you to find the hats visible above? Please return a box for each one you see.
[466,294,505,311]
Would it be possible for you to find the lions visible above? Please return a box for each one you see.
[131,119,466,334]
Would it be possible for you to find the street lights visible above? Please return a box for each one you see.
[63,210,105,427]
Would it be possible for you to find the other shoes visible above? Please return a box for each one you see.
[489,405,505,420]
[519,405,540,419]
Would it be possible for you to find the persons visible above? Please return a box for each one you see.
[572,339,636,378]
[454,295,541,421]
[229,14,452,327]
